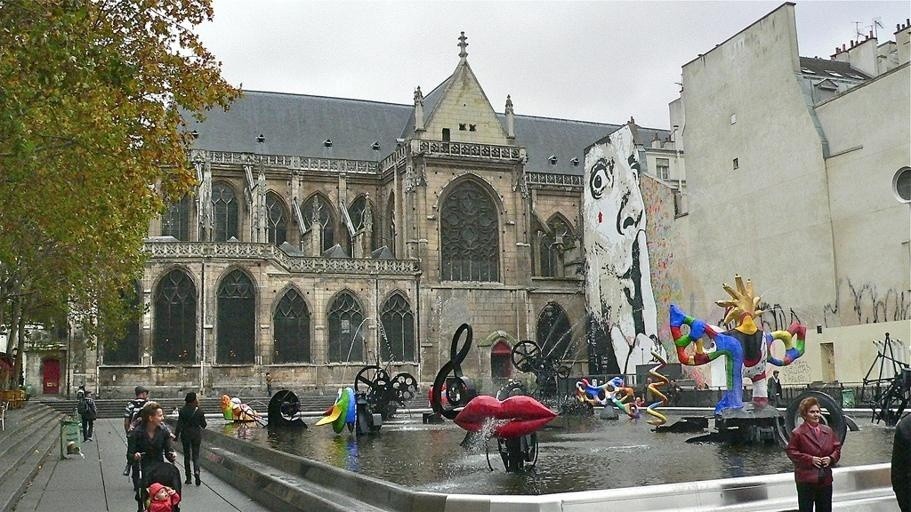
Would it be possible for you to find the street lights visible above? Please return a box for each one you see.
[196,253,212,399]
[368,269,384,379]
[412,270,424,385]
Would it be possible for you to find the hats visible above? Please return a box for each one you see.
[135,386,149,395]
[185,392,196,402]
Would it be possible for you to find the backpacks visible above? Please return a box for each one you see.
[130,400,148,422]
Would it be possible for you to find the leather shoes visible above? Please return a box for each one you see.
[185,471,200,486]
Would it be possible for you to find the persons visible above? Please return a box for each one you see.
[77,386,97,442]
[769,369,784,408]
[635,377,682,407]
[178,390,206,486]
[123,385,182,512]
[582,128,667,375]
[892,404,911,511]
[788,395,840,512]
[265,372,272,397]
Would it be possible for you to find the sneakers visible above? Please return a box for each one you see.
[83,437,93,442]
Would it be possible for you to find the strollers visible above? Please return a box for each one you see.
[136,451,182,512]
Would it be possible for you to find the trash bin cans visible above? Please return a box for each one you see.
[841,388,856,408]
[60,419,82,460]
